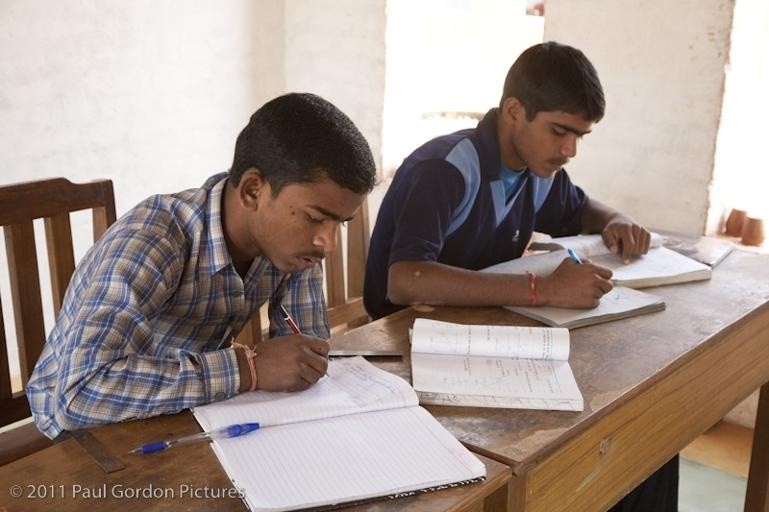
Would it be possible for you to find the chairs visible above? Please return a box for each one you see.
[0,179,118,470]
[232,196,371,351]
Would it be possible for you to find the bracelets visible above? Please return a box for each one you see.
[232,335,259,392]
[530,272,537,306]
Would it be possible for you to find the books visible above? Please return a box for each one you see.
[188,355,487,512]
[477,248,665,330]
[408,316,584,413]
[660,234,736,267]
[527,233,712,289]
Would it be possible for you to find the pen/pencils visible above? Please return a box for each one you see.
[567,248,583,264]
[127,422,259,457]
[277,304,330,378]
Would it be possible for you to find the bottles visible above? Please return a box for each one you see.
[726,209,764,247]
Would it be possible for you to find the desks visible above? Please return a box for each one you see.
[321,232,769,512]
[0,380,511,511]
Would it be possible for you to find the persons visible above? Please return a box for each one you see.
[25,92,374,446]
[362,41,679,512]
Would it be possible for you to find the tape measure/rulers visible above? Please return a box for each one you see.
[68,428,126,474]
[327,349,404,357]
